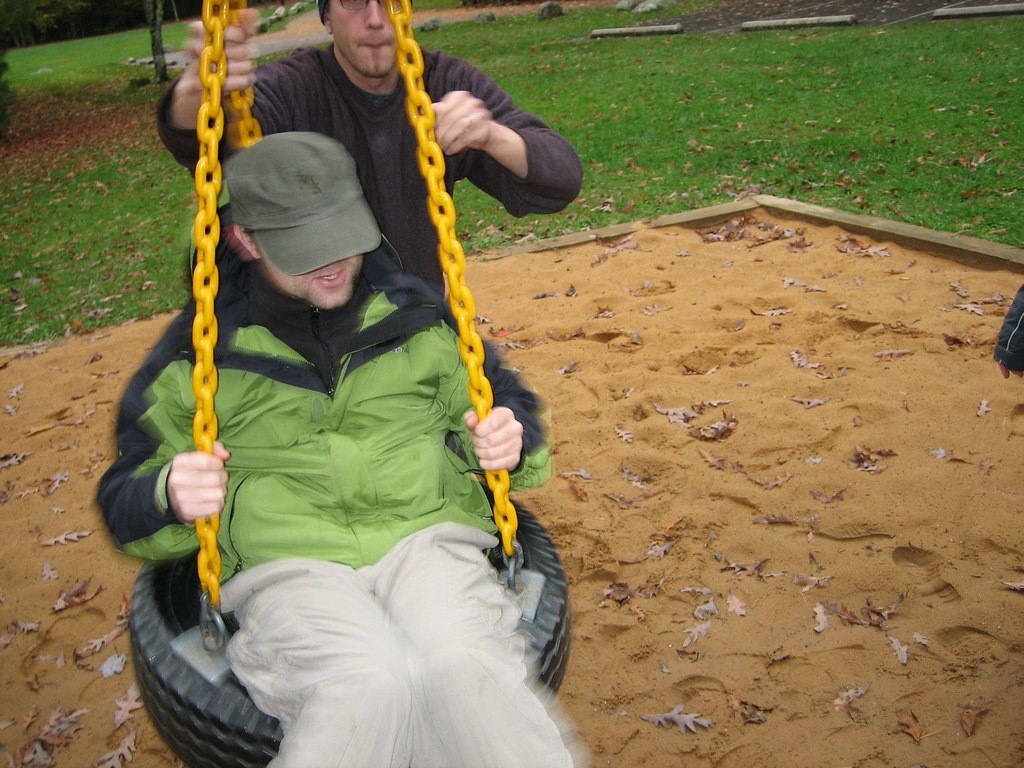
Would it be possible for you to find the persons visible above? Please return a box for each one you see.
[991,285,1023,377]
[159,0,583,308]
[98,125,594,765]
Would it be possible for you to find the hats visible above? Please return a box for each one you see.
[225,132,381,276]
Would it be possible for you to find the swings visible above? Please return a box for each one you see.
[123,0,576,768]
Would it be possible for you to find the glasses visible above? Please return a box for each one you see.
[340,0,386,10]
